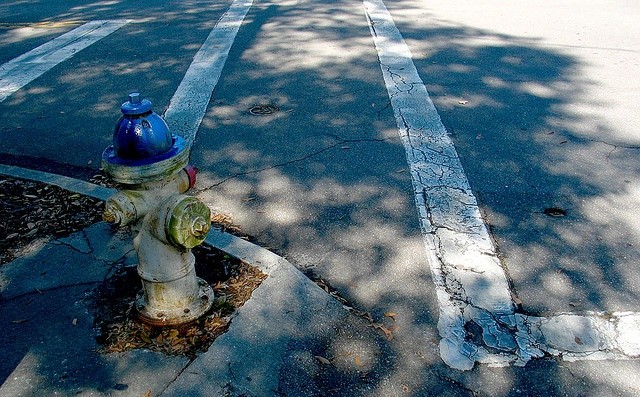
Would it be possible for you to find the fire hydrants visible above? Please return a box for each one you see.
[101,93,215,327]
